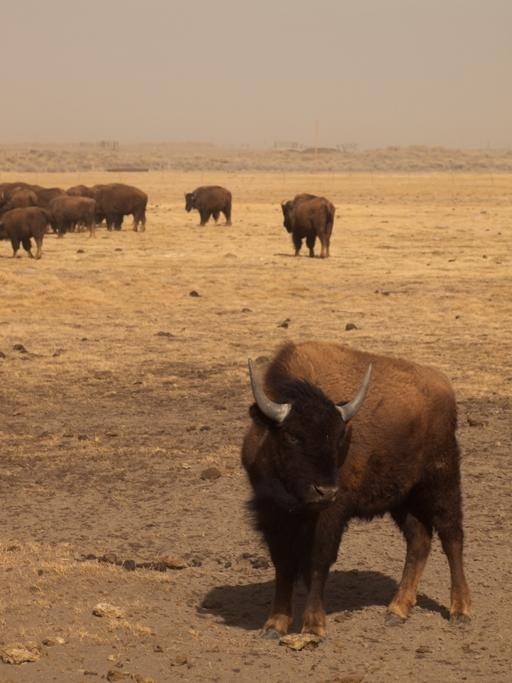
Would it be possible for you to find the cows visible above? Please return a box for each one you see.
[238,339,469,650]
[1,181,149,260]
[281,193,335,258]
[184,185,232,226]
